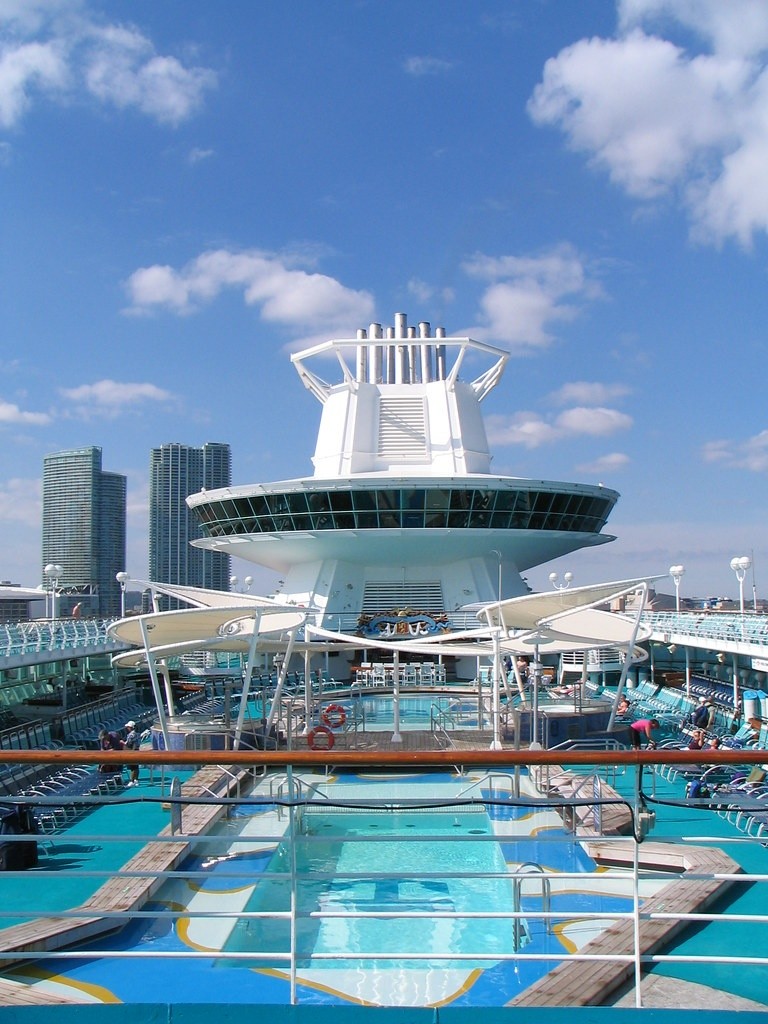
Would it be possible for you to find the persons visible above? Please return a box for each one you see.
[72,602,82,619]
[503,657,530,683]
[50,675,140,787]
[614,693,742,769]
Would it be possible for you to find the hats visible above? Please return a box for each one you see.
[125,721,136,728]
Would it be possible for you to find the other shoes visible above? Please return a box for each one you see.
[124,782,135,788]
[134,779,139,787]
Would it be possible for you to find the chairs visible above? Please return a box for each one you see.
[0,669,344,855]
[623,611,768,646]
[355,662,446,687]
[0,616,121,657]
[566,675,768,849]
[479,666,527,683]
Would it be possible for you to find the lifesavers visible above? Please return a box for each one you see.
[323,704,346,728]
[307,726,334,751]
